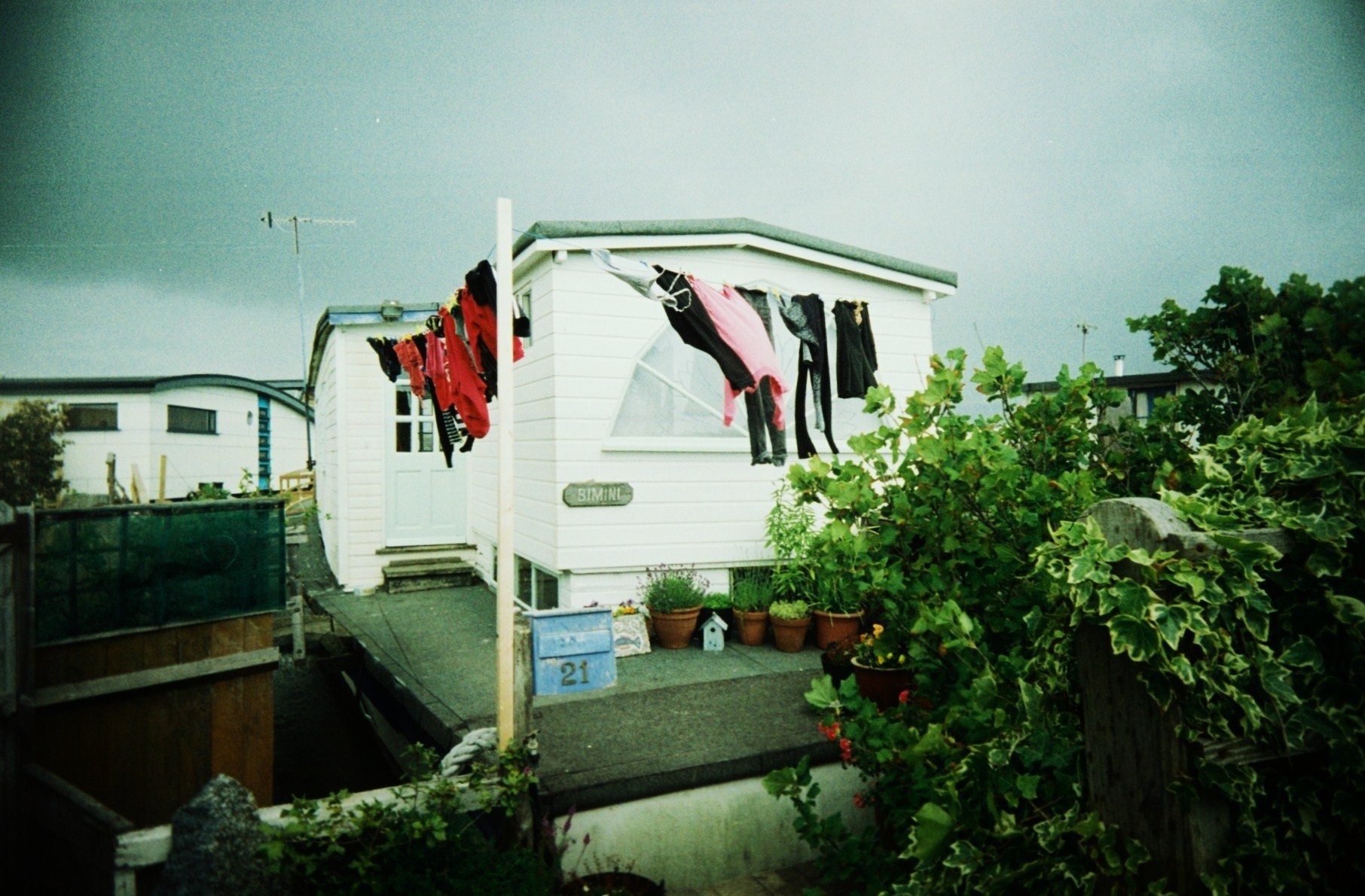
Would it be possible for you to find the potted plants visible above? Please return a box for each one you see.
[801,492,875,648]
[769,599,813,653]
[703,592,732,629]
[764,491,818,604]
[728,564,770,644]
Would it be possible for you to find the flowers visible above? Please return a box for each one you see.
[636,562,713,609]
[587,597,652,618]
[856,607,927,668]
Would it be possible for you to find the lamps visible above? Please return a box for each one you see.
[380,299,403,320]
[923,288,937,304]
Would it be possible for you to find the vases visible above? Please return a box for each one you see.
[611,610,652,658]
[848,648,927,711]
[653,603,703,650]
